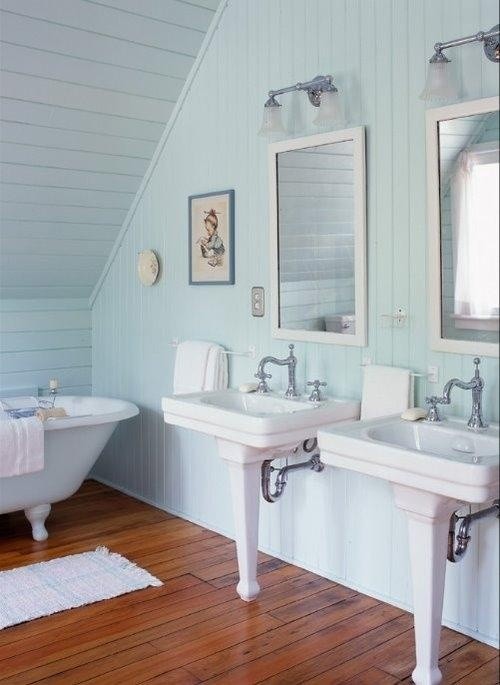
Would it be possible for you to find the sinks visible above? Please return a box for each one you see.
[161,390,360,447]
[316,415,500,504]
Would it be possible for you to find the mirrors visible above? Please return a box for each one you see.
[423,95,499,357]
[268,126,366,348]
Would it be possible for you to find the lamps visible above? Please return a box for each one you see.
[263,73,339,109]
[429,25,500,65]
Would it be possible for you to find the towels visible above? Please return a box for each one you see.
[358,363,414,421]
[1,416,45,479]
[172,340,229,392]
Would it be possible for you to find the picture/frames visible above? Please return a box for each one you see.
[187,189,235,286]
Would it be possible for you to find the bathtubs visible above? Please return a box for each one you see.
[0,396,140,542]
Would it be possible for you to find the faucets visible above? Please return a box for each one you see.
[254,344,299,397]
[442,358,488,429]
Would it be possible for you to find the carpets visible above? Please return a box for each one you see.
[1,545,165,633]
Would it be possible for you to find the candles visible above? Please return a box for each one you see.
[50,379,58,388]
